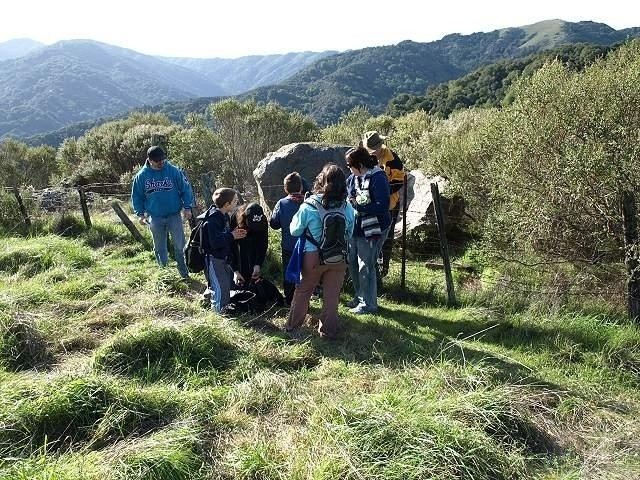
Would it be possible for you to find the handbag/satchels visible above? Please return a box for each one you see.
[220,277,284,316]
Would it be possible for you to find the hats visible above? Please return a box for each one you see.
[358,131,388,155]
[245,203,264,229]
[147,146,167,161]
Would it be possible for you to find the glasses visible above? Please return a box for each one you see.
[346,163,353,167]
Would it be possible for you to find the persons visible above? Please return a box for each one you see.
[205,187,247,314]
[131,146,194,279]
[270,171,310,306]
[345,146,393,314]
[230,203,268,287]
[359,131,405,289]
[285,163,355,340]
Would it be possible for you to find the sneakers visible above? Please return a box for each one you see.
[346,298,377,314]
[311,291,320,300]
[184,276,192,284]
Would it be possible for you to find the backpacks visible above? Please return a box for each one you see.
[183,220,205,274]
[304,197,349,266]
